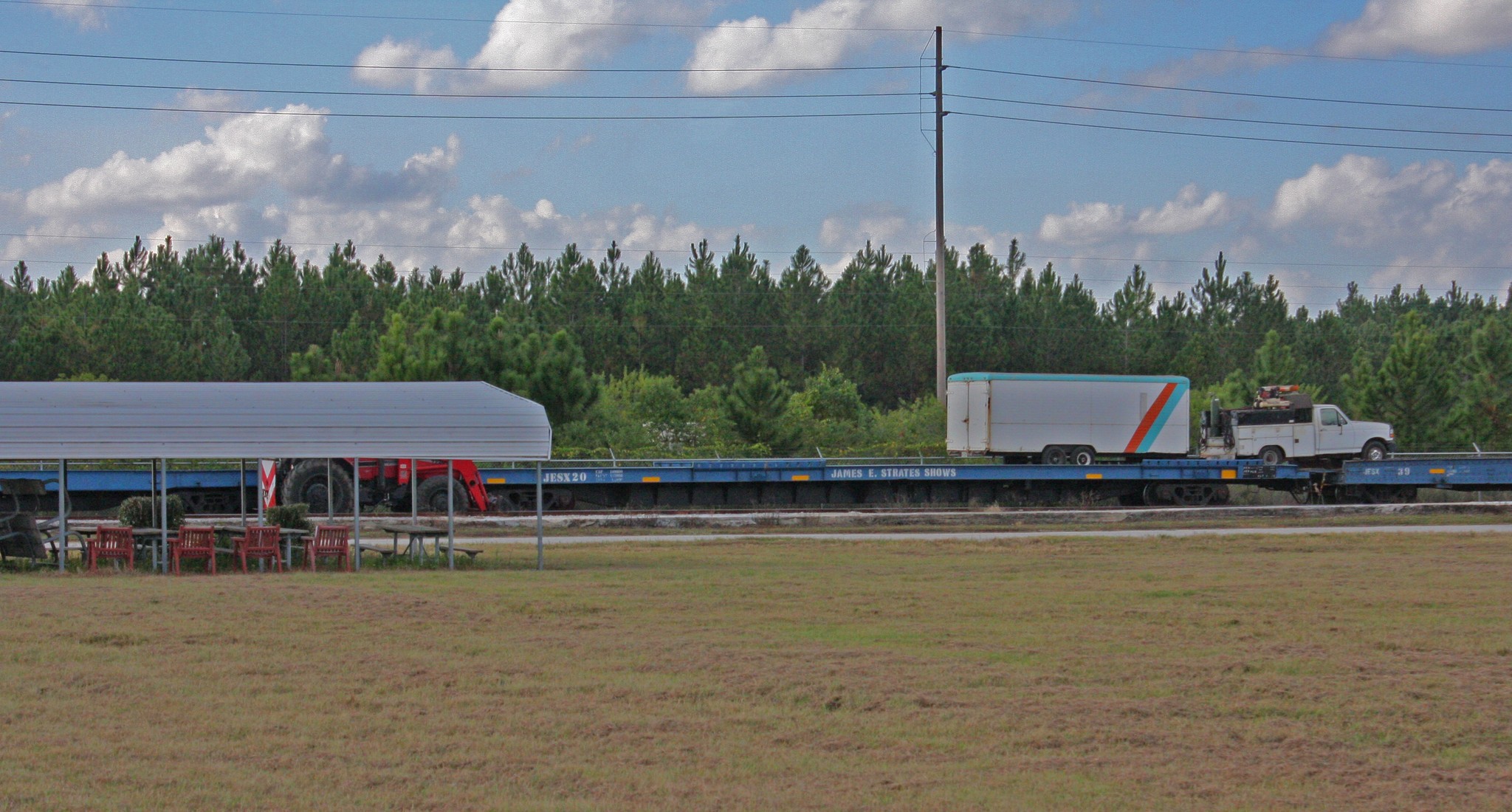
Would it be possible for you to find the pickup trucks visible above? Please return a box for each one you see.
[1197,384,1396,467]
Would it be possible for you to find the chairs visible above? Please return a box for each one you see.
[300,523,352,573]
[86,526,134,570]
[231,524,282,574]
[167,525,216,576]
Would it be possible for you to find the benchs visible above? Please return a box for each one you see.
[65,543,304,560]
[439,545,482,560]
[351,544,395,566]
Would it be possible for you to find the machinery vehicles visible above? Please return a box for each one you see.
[273,454,507,518]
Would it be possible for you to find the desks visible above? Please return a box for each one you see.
[383,524,456,566]
[72,526,163,565]
[170,527,224,560]
[223,526,309,565]
